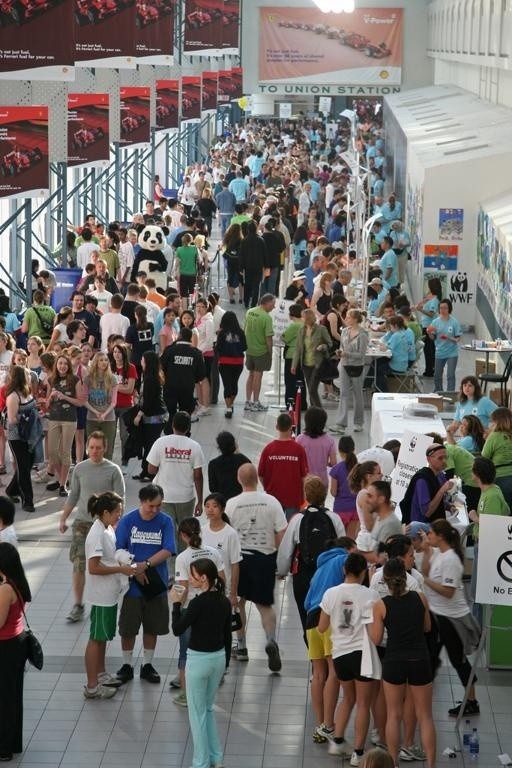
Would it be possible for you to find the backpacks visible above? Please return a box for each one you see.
[296,506,337,566]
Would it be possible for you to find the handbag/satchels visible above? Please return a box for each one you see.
[3,580,44,671]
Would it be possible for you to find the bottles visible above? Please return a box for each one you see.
[470,728,480,760]
[496,338,501,350]
[463,720,473,751]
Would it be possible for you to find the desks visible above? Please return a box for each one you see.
[369,392,448,477]
[460,343,512,374]
[365,347,392,393]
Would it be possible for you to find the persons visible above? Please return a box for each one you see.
[362,316,408,396]
[467,457,510,627]
[330,310,368,433]
[400,443,459,529]
[25,336,44,373]
[295,406,337,489]
[380,317,416,368]
[32,350,57,483]
[1,541,32,762]
[67,319,88,348]
[320,294,349,400]
[355,439,401,476]
[368,521,441,762]
[131,352,170,483]
[152,295,181,355]
[452,377,499,437]
[276,476,346,684]
[208,430,253,526]
[194,299,216,415]
[415,278,443,377]
[482,408,512,503]
[146,410,206,554]
[357,480,402,567]
[106,335,124,364]
[160,328,206,438]
[365,558,437,767]
[46,305,73,351]
[0,332,15,474]
[1,365,42,512]
[58,347,88,487]
[427,433,479,511]
[257,414,308,525]
[20,290,56,346]
[97,293,131,354]
[54,341,68,354]
[224,463,288,673]
[82,352,115,459]
[0,296,21,335]
[11,349,44,469]
[303,537,358,742]
[243,293,276,411]
[171,492,238,688]
[169,559,232,768]
[125,304,154,374]
[318,552,381,767]
[400,306,422,341]
[81,342,92,370]
[0,496,18,549]
[371,305,395,331]
[446,415,485,455]
[179,310,199,421]
[279,304,304,412]
[291,309,332,408]
[115,483,177,684]
[57,431,126,624]
[42,356,82,497]
[174,516,227,709]
[84,294,104,346]
[206,290,225,405]
[1,117,422,309]
[419,519,481,716]
[356,746,396,768]
[329,435,360,539]
[214,311,247,418]
[0,316,16,351]
[157,308,178,355]
[425,298,462,391]
[83,492,137,700]
[68,291,97,348]
[111,345,136,465]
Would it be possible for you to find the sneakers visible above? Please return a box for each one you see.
[83,684,117,699]
[23,504,35,512]
[172,693,187,707]
[321,391,328,399]
[250,400,268,411]
[230,645,249,661]
[0,464,7,474]
[328,739,353,756]
[370,728,380,744]
[328,424,345,434]
[65,604,85,621]
[132,474,142,479]
[36,461,49,470]
[34,472,55,482]
[190,416,199,423]
[312,727,329,743]
[244,400,252,410]
[97,672,122,687]
[6,489,19,502]
[46,480,60,491]
[59,486,68,496]
[115,664,134,683]
[353,424,363,432]
[327,393,340,402]
[225,412,232,418]
[169,675,180,687]
[141,477,151,482]
[349,751,367,766]
[197,406,208,416]
[264,638,282,671]
[448,698,480,715]
[316,723,335,743]
[122,465,127,474]
[375,743,388,752]
[399,744,427,761]
[140,663,160,683]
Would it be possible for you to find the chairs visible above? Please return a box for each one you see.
[391,341,425,392]
[478,353,512,406]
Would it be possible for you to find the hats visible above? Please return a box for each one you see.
[367,277,382,285]
[369,260,380,266]
[292,271,306,281]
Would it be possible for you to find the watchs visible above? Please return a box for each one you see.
[143,559,151,569]
